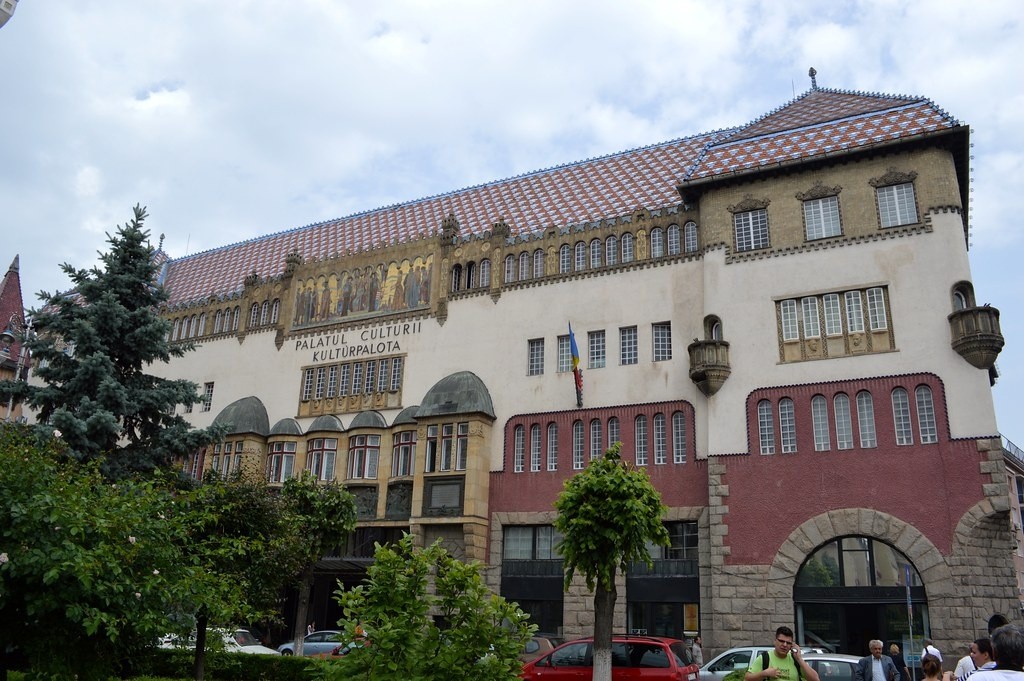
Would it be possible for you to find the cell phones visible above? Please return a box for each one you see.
[790,647,797,653]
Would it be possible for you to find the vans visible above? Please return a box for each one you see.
[699,645,812,681]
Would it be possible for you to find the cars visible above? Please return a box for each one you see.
[514,634,699,681]
[165,626,281,656]
[279,629,362,659]
[798,654,869,681]
[310,638,372,660]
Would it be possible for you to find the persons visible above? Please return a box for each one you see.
[690,636,705,668]
[854,638,900,681]
[919,653,954,681]
[953,638,994,681]
[954,640,984,678]
[964,624,1024,681]
[886,642,912,681]
[922,638,945,676]
[745,626,820,681]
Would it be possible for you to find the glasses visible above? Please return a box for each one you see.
[776,638,792,645]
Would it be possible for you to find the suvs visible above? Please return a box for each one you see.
[506,636,567,666]
